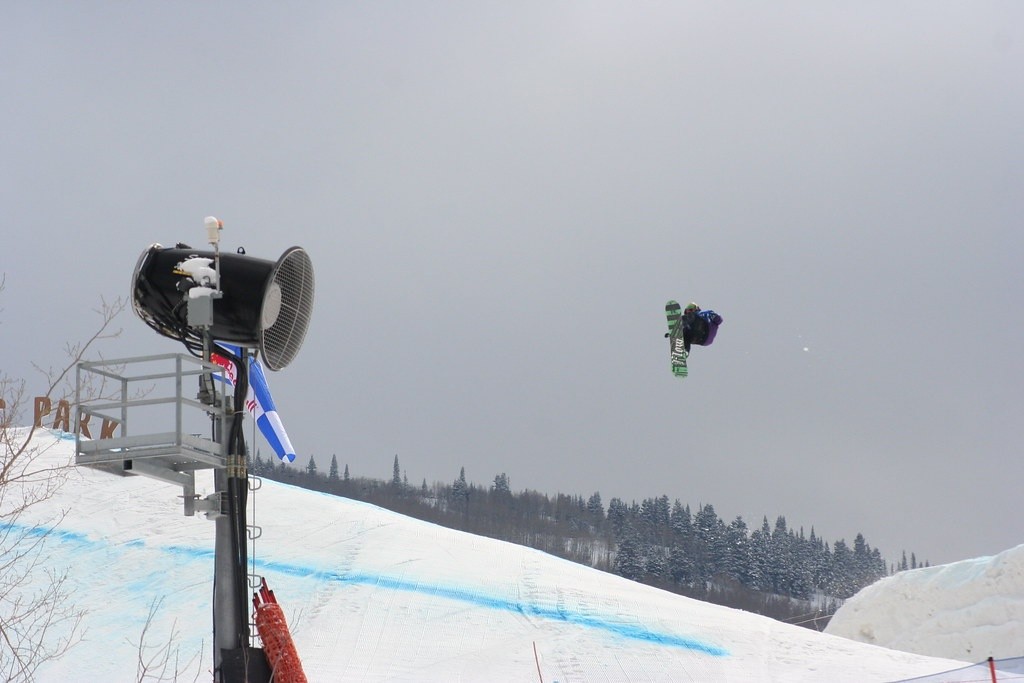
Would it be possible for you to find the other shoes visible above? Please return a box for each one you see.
[686,351,688,356]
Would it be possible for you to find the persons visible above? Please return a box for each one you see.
[664,302,723,358]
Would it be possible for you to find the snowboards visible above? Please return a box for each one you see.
[663,298,689,381]
